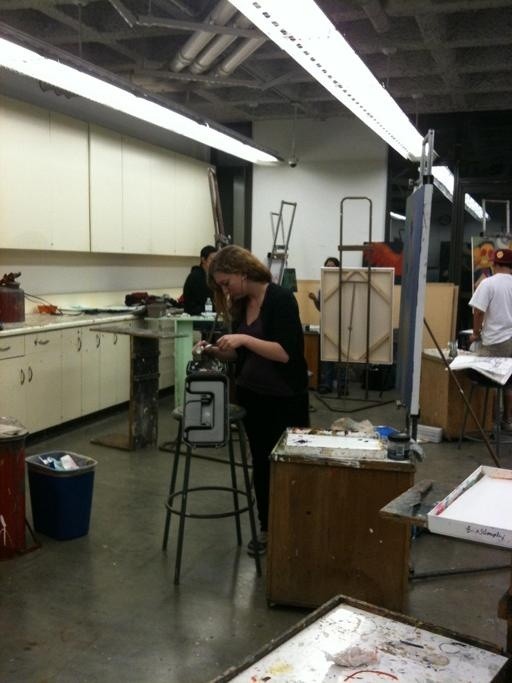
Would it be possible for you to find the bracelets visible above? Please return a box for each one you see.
[473,328,482,335]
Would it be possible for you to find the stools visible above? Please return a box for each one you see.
[160,401,265,586]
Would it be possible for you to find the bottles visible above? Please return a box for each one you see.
[205,297,213,312]
[388,432,409,460]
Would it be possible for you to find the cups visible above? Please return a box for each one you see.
[448,341,459,357]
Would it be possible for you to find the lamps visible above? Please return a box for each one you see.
[422,162,497,223]
[236,0,441,167]
[0,0,288,168]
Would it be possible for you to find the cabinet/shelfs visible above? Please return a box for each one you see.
[87,121,217,256]
[63,319,133,425]
[265,425,416,618]
[0,92,92,255]
[0,330,64,437]
[420,348,494,442]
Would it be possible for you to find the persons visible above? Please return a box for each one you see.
[183,246,216,316]
[474,241,496,292]
[468,249,512,432]
[192,245,310,555]
[308,257,349,397]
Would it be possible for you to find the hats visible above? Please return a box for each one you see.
[488,249,511,264]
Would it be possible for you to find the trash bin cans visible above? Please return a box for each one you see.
[0,424,29,560]
[25,450,98,541]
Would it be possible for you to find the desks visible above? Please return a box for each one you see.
[147,314,228,409]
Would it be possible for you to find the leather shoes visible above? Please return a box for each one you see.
[245,531,268,554]
